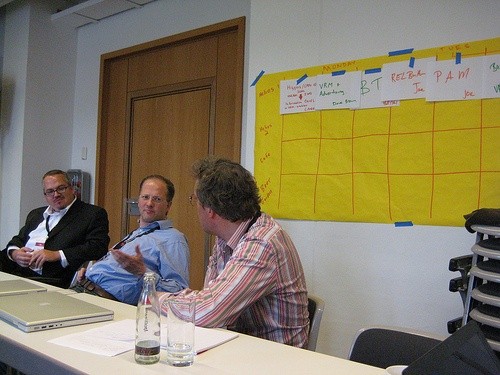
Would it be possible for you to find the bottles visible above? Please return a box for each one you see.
[135,271,161,365]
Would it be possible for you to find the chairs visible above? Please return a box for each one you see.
[307,295,325,352]
[347,208,500,370]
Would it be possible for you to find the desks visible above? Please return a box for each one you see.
[0,270,386,375]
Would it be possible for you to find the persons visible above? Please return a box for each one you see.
[0,170,110,290]
[70,175,191,306]
[142,160,309,349]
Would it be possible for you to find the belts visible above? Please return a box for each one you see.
[80,278,120,303]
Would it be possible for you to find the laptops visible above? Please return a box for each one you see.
[0,291,115,333]
[0,279,46,296]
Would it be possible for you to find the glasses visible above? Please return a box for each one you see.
[44,185,69,195]
[190,193,199,207]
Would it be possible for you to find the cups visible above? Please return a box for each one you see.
[166,297,195,367]
